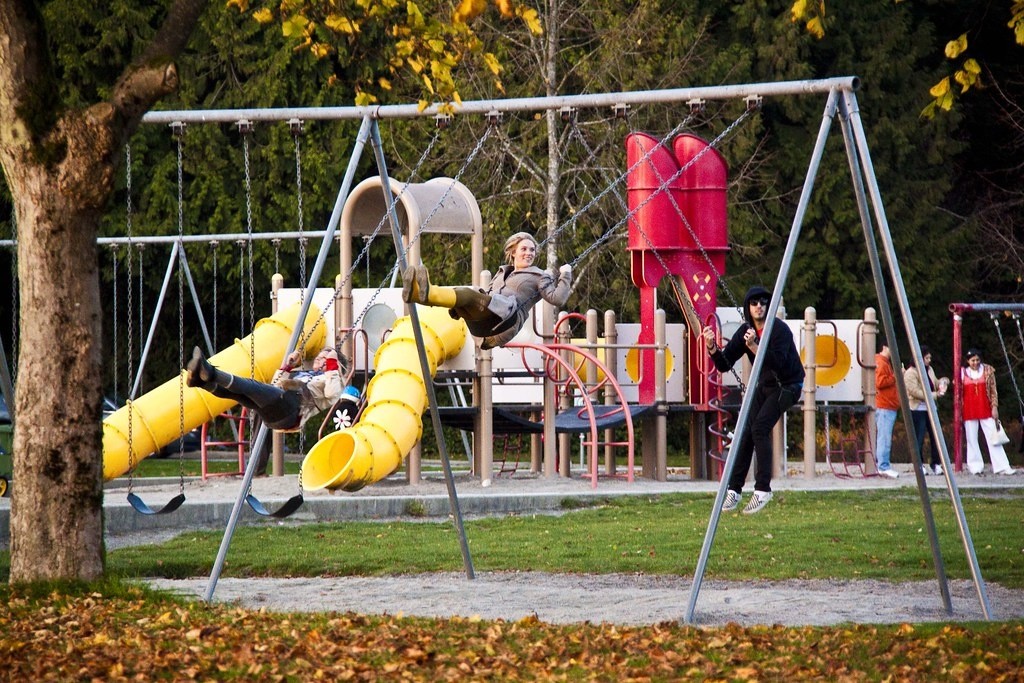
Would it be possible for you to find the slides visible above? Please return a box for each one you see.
[297,305,468,493]
[103,302,329,482]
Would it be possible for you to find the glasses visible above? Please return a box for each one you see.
[749,297,769,306]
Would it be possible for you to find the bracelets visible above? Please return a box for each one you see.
[707,344,714,350]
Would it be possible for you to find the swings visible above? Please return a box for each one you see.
[0,199,18,456]
[471,110,760,348]
[124,118,186,516]
[259,111,502,430]
[334,235,372,288]
[990,310,1024,422]
[209,238,246,354]
[109,242,145,411]
[566,95,799,413]
[233,118,305,518]
[271,237,304,305]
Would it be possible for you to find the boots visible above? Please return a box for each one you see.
[417,265,457,308]
[402,266,432,307]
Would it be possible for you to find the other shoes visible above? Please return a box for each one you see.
[934,463,945,475]
[999,468,1017,475]
[922,466,929,476]
[878,469,898,478]
[188,363,202,387]
[193,346,216,382]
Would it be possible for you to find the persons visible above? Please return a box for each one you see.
[402,232,572,351]
[961,349,1017,475]
[331,385,361,431]
[874,338,906,478]
[903,346,950,475]
[185,346,348,430]
[702,285,806,514]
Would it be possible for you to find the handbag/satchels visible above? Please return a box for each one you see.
[991,418,1010,445]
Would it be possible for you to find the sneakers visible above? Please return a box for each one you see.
[743,489,773,514]
[722,489,742,511]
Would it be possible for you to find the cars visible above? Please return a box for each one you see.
[1,392,201,458]
